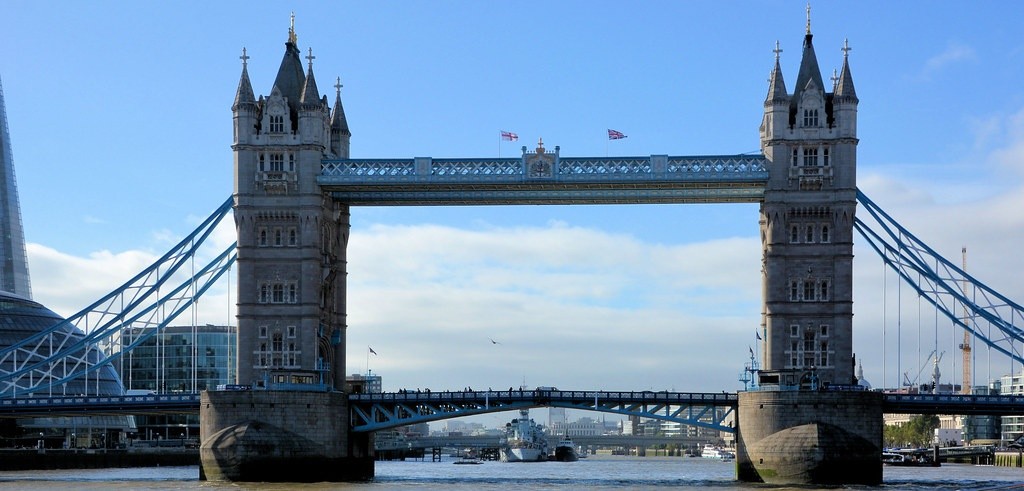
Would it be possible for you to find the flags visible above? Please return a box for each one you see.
[501,131,518,141]
[369,348,376,355]
[607,129,626,140]
[756,332,761,339]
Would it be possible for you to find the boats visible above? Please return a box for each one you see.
[553,416,578,461]
[499,376,548,462]
[579,451,589,458]
[882,458,941,468]
[721,455,731,462]
[701,444,736,458]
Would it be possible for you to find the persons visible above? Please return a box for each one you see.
[519,386,522,391]
[489,387,492,391]
[509,387,513,391]
[465,386,472,392]
[416,388,421,392]
[398,388,407,393]
[89,440,96,448]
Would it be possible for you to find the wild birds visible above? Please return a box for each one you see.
[486,335,503,347]
